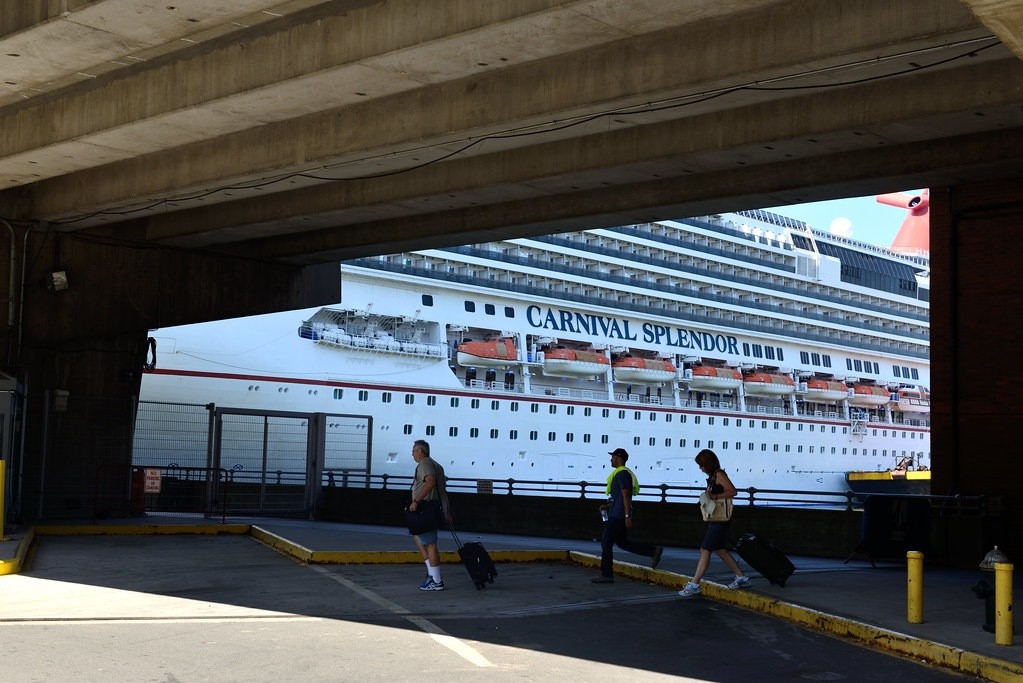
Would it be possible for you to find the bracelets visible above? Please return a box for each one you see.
[414,500,419,504]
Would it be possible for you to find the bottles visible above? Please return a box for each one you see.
[601,508,608,522]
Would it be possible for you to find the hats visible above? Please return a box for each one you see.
[607,448,629,461]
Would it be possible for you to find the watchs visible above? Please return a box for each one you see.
[625,515,629,518]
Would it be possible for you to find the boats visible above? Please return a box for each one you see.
[847,456,935,514]
[458,337,891,406]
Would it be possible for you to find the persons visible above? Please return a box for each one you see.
[409,440,446,591]
[589,448,663,584]
[678,449,751,596]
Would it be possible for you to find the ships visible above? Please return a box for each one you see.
[132,204,937,507]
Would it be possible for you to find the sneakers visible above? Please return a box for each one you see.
[678,581,703,597]
[419,576,433,589]
[728,575,752,590]
[419,576,445,591]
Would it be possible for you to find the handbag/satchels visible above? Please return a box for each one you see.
[702,469,732,522]
[403,500,446,536]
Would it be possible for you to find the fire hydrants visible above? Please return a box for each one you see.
[973,544,1014,635]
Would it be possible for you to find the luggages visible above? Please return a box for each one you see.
[725,518,797,588]
[447,522,498,591]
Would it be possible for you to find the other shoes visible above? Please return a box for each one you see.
[651,546,664,569]
[589,575,615,583]
